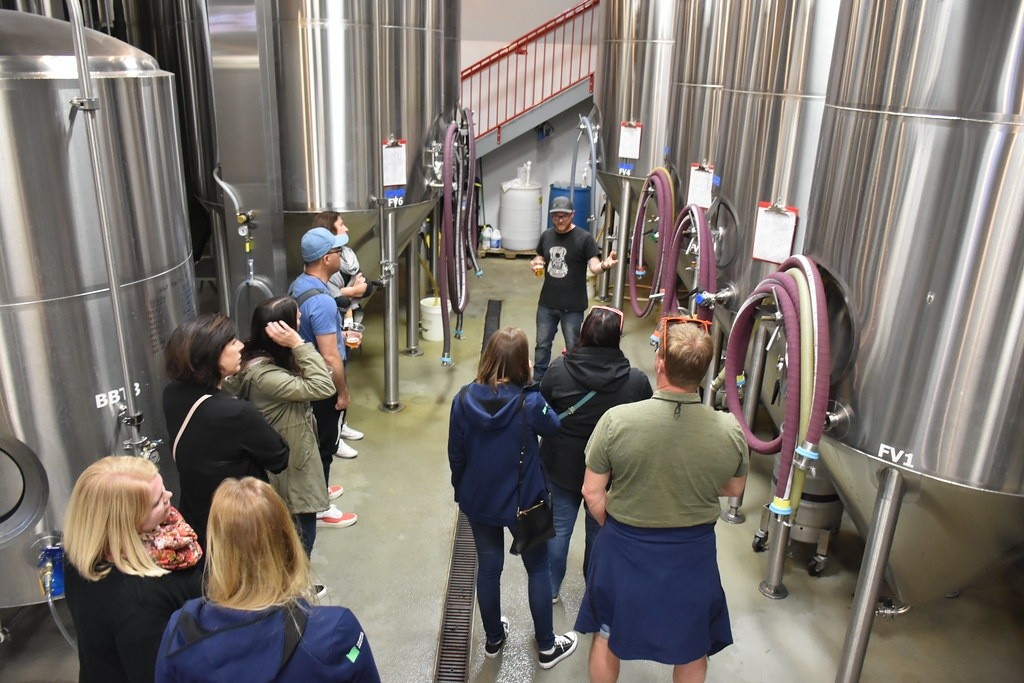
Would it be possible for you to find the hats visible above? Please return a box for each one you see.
[550,196,573,213]
[301,228,350,262]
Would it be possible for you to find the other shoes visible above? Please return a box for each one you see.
[309,585,326,602]
[553,596,559,604]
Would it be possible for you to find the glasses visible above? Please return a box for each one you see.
[589,305,625,335]
[552,213,572,221]
[326,246,343,257]
[662,316,713,365]
[332,221,347,228]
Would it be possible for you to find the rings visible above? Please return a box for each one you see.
[281,330,286,334]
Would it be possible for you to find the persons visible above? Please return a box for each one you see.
[448,325,578,670]
[303,209,373,460]
[288,227,358,529]
[154,475,382,683]
[530,196,620,391]
[60,454,206,683]
[235,293,337,601]
[573,310,750,683]
[162,310,290,572]
[537,304,653,606]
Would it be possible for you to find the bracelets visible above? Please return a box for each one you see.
[601,260,608,271]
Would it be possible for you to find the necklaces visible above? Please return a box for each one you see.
[651,396,701,419]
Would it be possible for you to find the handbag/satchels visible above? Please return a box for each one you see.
[509,498,556,556]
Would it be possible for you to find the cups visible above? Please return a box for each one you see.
[534,261,545,277]
[345,322,365,348]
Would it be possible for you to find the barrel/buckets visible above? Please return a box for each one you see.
[420,296,452,341]
[480,225,501,251]
[498,180,542,251]
[547,183,591,232]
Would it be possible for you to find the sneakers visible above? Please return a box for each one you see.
[336,439,358,458]
[340,424,364,441]
[315,506,357,529]
[327,485,344,502]
[539,631,578,669]
[485,616,510,658]
